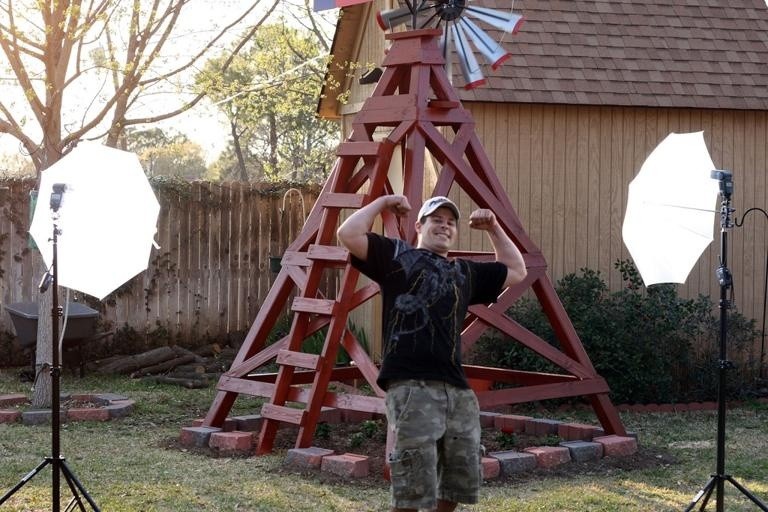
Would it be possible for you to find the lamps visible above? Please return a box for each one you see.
[686,168,767,512]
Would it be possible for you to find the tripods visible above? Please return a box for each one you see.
[680,196,768,512]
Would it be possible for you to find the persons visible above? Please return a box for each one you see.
[335,194,529,511]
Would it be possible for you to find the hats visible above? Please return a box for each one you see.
[418,196,460,223]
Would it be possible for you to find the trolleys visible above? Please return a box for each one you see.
[3,300,113,385]
